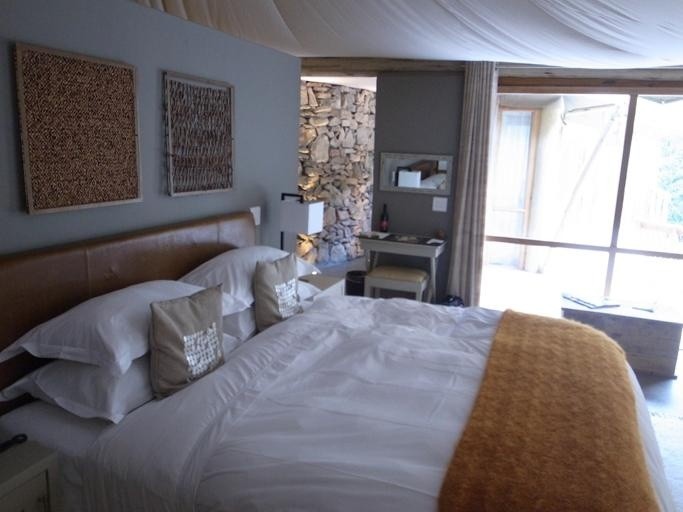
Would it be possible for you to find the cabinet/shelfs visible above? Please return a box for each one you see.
[560,297,683,379]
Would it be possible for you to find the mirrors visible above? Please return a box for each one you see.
[379,153,453,197]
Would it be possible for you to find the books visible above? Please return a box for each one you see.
[562,291,621,310]
[572,295,620,307]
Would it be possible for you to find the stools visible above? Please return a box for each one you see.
[363,265,431,303]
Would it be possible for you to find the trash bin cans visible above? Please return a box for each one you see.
[346,270,367,296]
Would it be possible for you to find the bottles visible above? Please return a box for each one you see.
[378,203,389,232]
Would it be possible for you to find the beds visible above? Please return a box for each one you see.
[1,210,673,512]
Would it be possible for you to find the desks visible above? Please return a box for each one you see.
[357,232,449,304]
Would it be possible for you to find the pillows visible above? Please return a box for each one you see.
[179,246,320,315]
[149,285,226,401]
[252,251,305,333]
[3,331,240,423]
[1,279,249,375]
[222,280,320,341]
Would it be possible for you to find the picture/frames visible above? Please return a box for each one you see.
[162,68,236,197]
[12,38,143,216]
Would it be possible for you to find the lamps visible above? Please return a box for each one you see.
[276,192,325,248]
